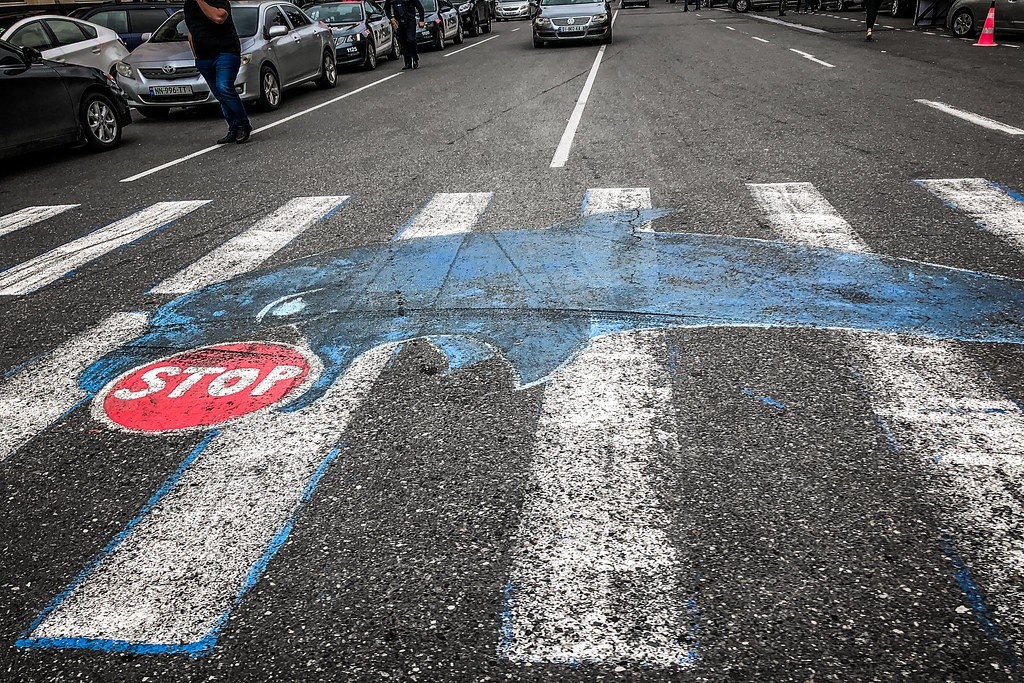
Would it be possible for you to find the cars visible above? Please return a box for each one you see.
[669,0,1024,39]
[495,0,536,22]
[621,0,649,9]
[115,0,338,118]
[375,0,464,51]
[297,0,401,71]
[0,12,131,83]
[528,0,612,49]
[0,38,134,161]
[450,0,492,37]
[0,0,186,52]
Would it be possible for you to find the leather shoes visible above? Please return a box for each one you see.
[217,130,236,144]
[236,125,252,143]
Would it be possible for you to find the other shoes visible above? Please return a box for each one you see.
[866,32,871,40]
[413,59,418,69]
[402,63,411,70]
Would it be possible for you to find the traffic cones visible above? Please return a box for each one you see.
[972,7,999,46]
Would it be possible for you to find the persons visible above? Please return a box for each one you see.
[778,0,818,16]
[866,0,882,41]
[384,0,425,70]
[183,0,252,144]
